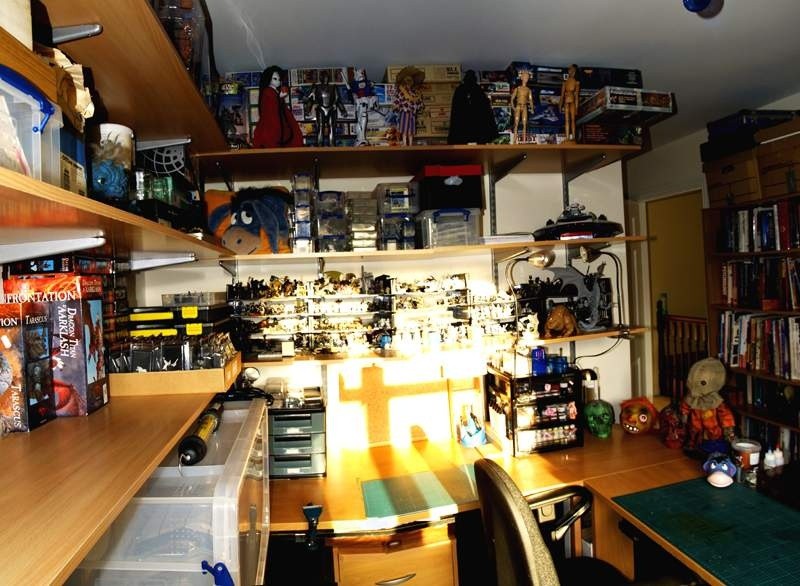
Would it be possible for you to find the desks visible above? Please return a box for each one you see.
[270,424,800,586]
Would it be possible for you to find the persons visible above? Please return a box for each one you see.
[305,70,348,147]
[559,64,580,145]
[585,399,615,439]
[509,64,534,145]
[658,402,689,450]
[620,395,659,435]
[250,65,305,147]
[393,67,426,145]
[90,131,132,208]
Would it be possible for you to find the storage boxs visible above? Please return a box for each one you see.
[699,109,800,207]
[0,63,678,586]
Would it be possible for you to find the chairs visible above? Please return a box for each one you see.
[474,459,638,586]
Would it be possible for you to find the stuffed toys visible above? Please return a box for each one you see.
[208,185,297,255]
[703,454,737,488]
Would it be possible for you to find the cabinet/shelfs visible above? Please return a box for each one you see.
[0,0,236,586]
[700,193,800,433]
[332,531,459,586]
[194,0,650,586]
